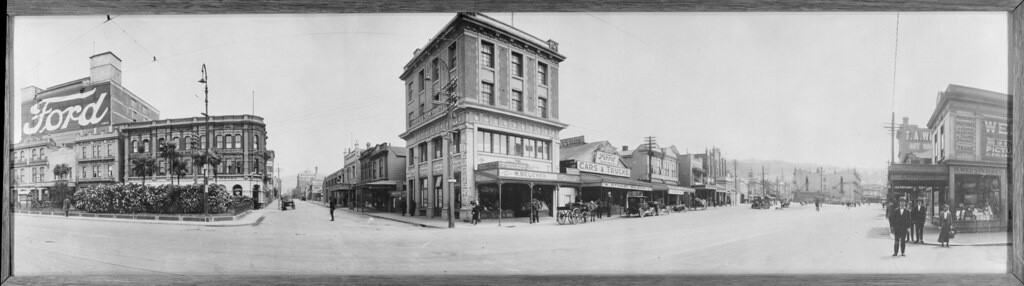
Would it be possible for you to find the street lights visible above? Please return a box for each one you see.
[424,56,455,228]
[198,63,209,216]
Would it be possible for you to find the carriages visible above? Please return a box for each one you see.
[556,200,599,225]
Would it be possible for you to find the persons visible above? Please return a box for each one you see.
[471,197,539,225]
[400,197,415,216]
[62,194,70,217]
[937,203,952,247]
[912,197,926,244]
[884,199,914,242]
[326,196,335,221]
[577,197,611,224]
[889,197,911,257]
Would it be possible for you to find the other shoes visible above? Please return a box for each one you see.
[915,240,918,243]
[902,254,905,256]
[893,254,897,256]
[941,244,944,246]
[331,220,334,221]
[947,244,949,247]
[906,240,909,242]
[921,241,924,244]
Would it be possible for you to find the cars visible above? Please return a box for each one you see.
[626,196,656,217]
[751,196,763,209]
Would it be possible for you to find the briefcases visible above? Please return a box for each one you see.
[949,229,954,238]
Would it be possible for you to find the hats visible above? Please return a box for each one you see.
[942,204,949,208]
[899,197,906,201]
[591,201,593,203]
[917,197,922,201]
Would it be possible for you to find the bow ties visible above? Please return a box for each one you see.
[900,208,904,209]
